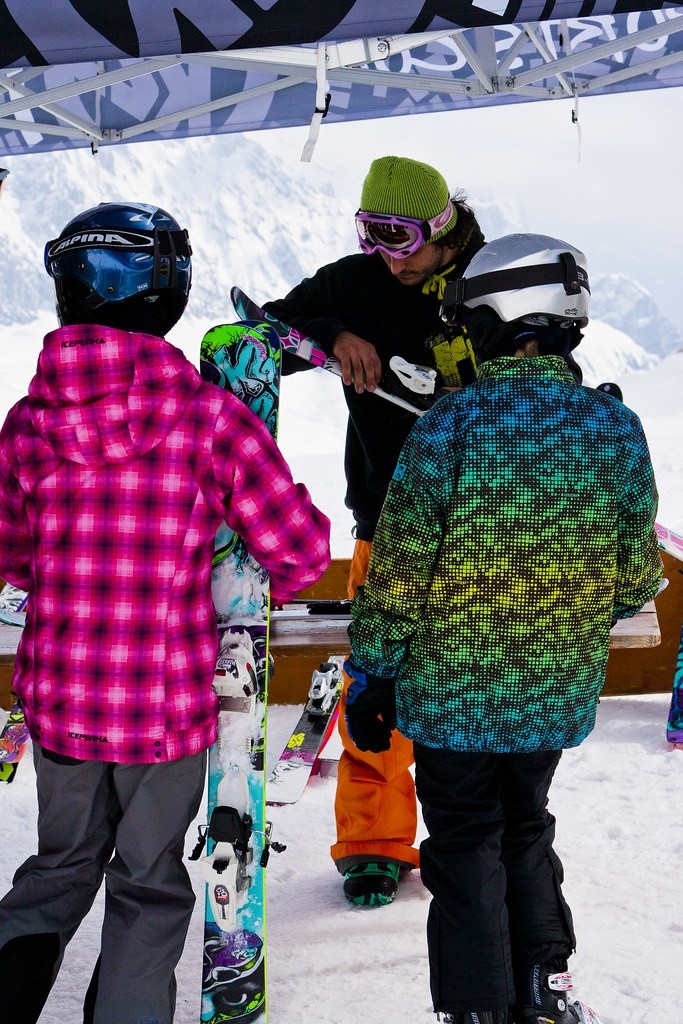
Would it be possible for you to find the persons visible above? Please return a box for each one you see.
[343,233,666,1024]
[255,156,486,907]
[0,203,329,1024]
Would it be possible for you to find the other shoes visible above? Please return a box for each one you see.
[666,727,683,751]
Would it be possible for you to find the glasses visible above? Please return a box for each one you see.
[354,210,432,259]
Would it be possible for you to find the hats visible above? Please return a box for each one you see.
[361,156,458,247]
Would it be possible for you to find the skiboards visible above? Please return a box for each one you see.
[0,654,352,807]
[230,284,683,748]
[198,319,284,1024]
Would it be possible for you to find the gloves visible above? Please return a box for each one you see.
[342,657,399,753]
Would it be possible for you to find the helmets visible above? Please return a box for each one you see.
[456,233,590,329]
[44,202,192,333]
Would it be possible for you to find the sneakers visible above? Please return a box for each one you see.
[516,953,602,1024]
[342,860,400,906]
